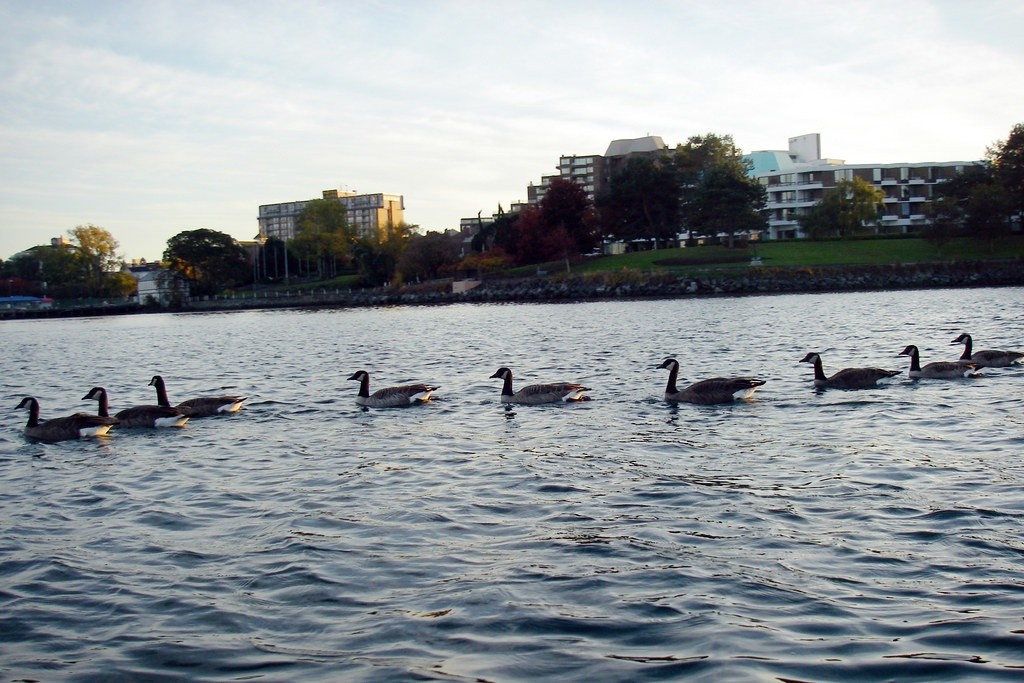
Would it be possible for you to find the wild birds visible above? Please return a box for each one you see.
[656,359,767,404]
[951,333,1024,368]
[898,344,981,380]
[13,375,250,446]
[347,370,442,406]
[489,367,594,404]
[799,351,903,390]
